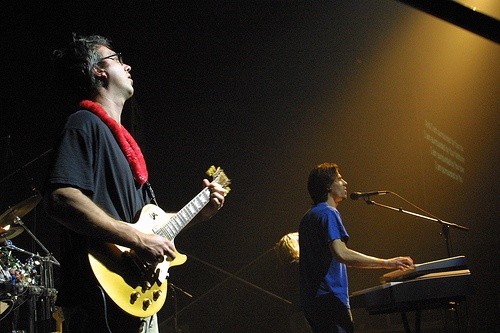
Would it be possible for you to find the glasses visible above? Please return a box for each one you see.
[101,52,123,63]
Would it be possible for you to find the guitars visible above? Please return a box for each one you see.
[87,165,231,317]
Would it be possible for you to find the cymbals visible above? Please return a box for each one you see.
[1,193,42,223]
[1,226,24,242]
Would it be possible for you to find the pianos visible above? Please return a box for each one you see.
[348,268,474,316]
[379,255,466,283]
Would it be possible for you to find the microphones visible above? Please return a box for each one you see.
[350,191,390,200]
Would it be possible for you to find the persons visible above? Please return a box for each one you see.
[40,31,232,333]
[276,230,314,333]
[298,161,414,332]
